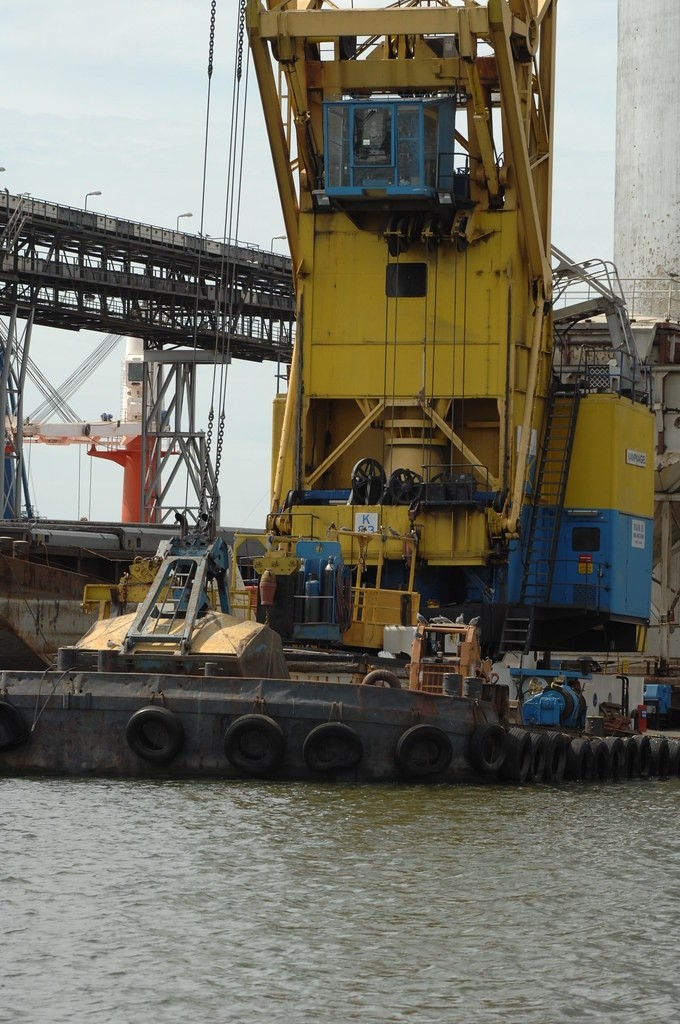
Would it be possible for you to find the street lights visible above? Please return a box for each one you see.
[175,213,195,232]
[271,235,287,253]
[84,191,102,211]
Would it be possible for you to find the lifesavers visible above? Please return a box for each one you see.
[500,726,680,787]
[468,722,509,775]
[302,721,364,774]
[0,700,29,754]
[361,668,402,688]
[126,705,186,765]
[393,723,454,779]
[223,713,285,775]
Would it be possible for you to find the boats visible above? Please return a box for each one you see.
[0,0,677,786]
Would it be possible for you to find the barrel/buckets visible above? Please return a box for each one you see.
[58,645,78,671]
[586,716,604,736]
[98,649,120,673]
[462,677,482,699]
[442,673,462,697]
[204,662,218,677]
